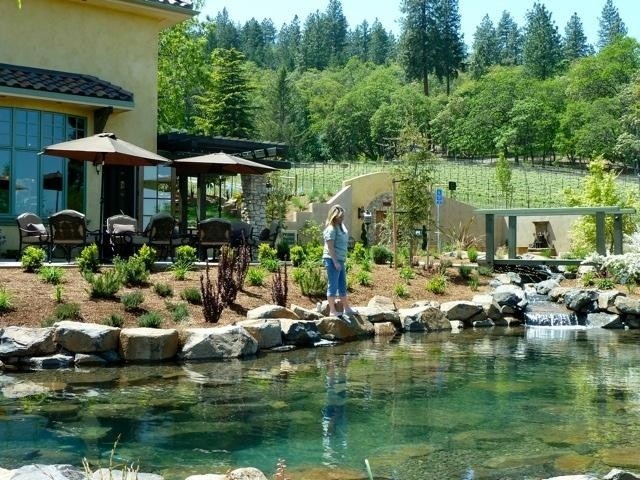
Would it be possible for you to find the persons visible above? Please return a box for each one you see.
[321,350,353,479]
[322,204,362,318]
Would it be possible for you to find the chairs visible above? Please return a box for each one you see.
[15,208,280,265]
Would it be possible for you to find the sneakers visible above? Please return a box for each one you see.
[329,312,341,317]
[345,307,358,315]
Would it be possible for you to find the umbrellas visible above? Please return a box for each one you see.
[164,148,282,219]
[38,132,169,247]
